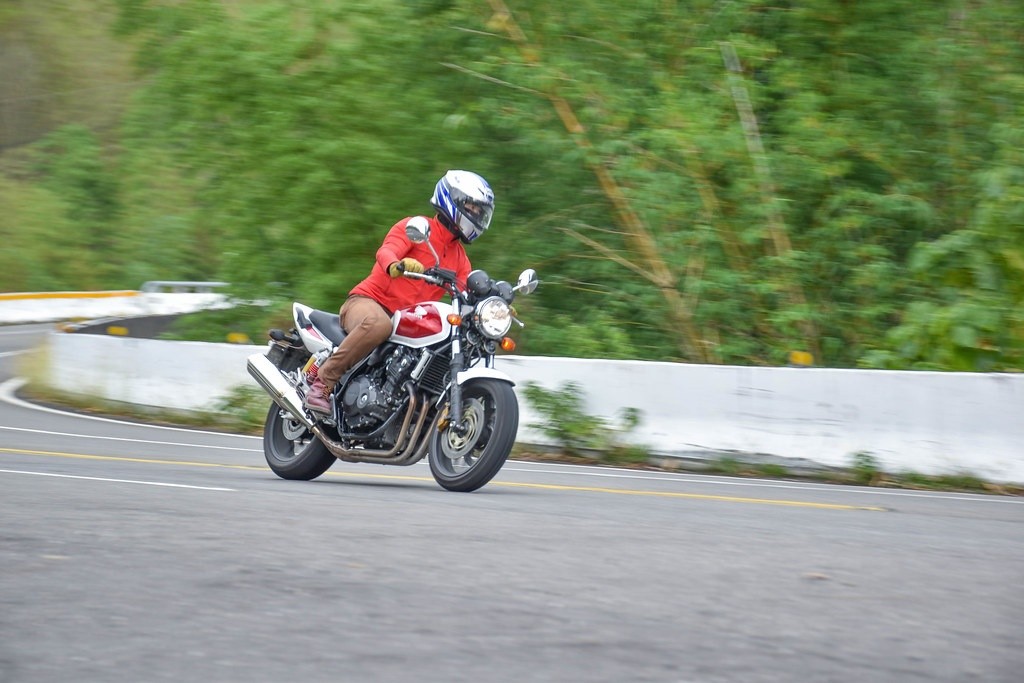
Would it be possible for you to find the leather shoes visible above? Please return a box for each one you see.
[303,377,331,414]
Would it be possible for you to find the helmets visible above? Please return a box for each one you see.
[431,169,494,244]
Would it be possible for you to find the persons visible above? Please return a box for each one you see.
[305,170,518,415]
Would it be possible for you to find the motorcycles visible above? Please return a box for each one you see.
[247,216,541,492]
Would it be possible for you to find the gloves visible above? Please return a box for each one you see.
[390,258,424,279]
[510,306,517,317]
